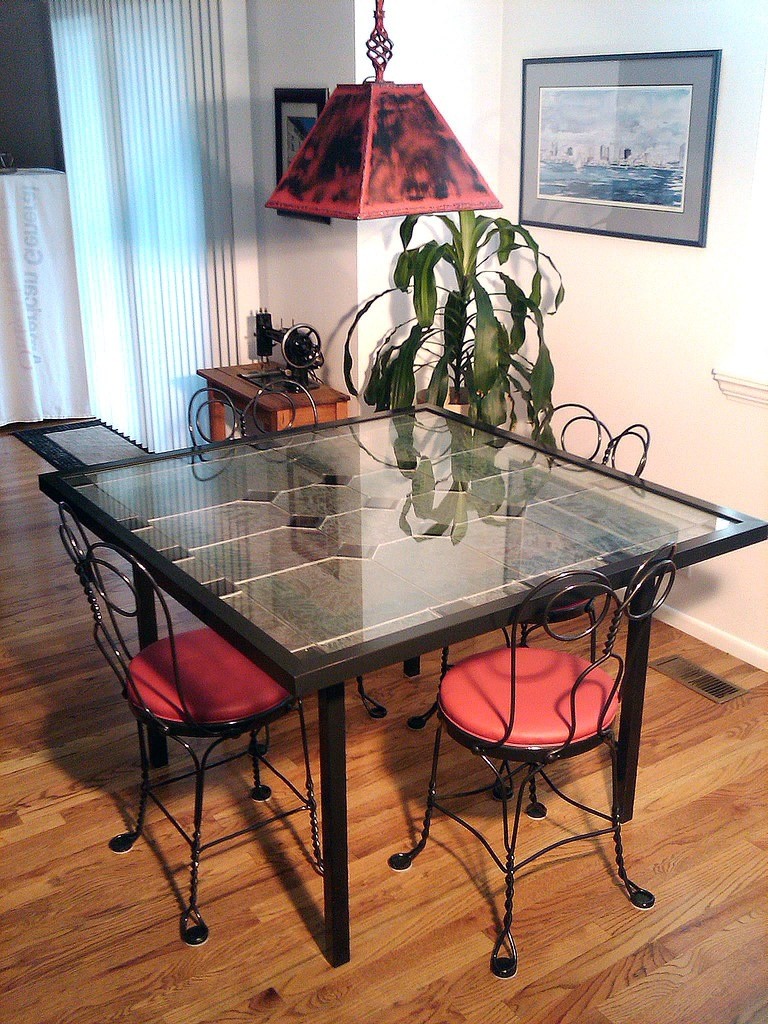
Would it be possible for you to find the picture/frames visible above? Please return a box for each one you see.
[274,87,329,225]
[518,48,723,248]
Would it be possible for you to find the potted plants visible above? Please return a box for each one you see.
[342,210,565,450]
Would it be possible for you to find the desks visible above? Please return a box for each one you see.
[36,403,768,968]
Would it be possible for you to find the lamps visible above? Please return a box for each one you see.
[264,0,503,220]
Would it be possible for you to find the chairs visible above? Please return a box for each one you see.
[58,502,323,947]
[388,541,677,980]
[406,404,650,731]
[188,380,388,720]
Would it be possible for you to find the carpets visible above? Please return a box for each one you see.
[7,419,155,471]
